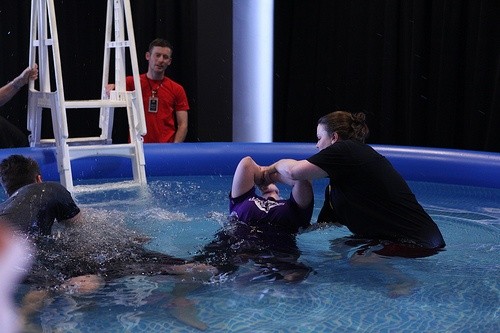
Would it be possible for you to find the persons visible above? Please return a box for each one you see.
[0,63,38,105]
[229,156,314,232]
[106,39,190,143]
[0,156,81,243]
[259,111,445,246]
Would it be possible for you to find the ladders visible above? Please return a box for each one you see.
[26,0,148,207]
